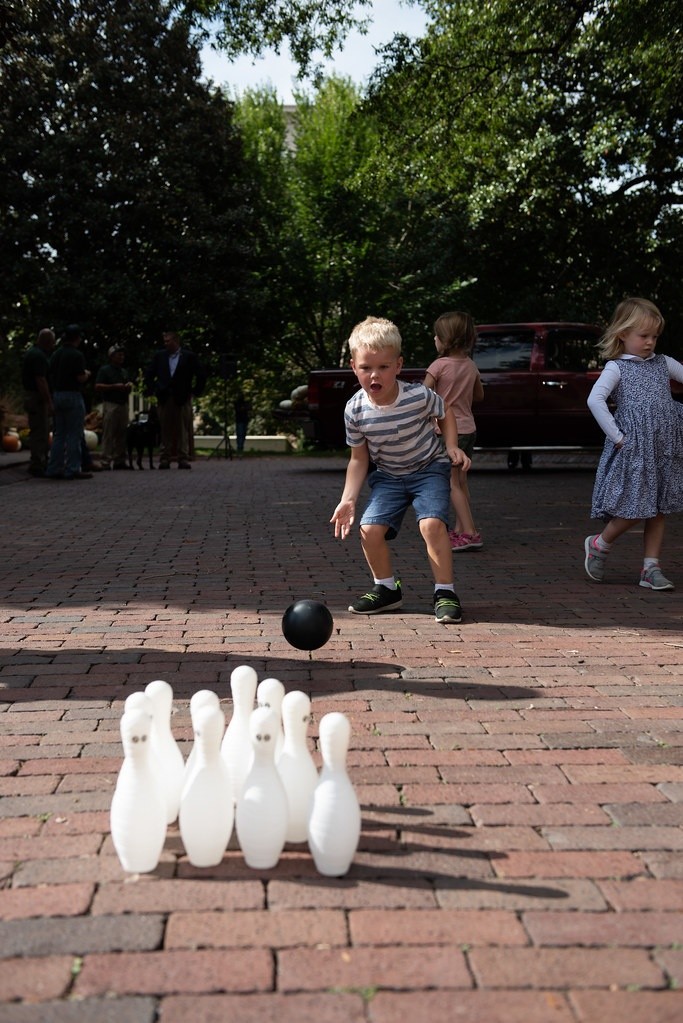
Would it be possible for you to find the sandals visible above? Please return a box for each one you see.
[448,530,484,550]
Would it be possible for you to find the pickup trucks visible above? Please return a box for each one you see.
[308,322,683,470]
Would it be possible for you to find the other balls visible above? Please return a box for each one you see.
[281,599,333,651]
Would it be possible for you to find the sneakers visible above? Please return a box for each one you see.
[639,563,675,590]
[585,533,610,581]
[348,581,403,615]
[433,588,461,623]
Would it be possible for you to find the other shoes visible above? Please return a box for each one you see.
[178,461,191,469]
[160,460,170,469]
[104,464,111,470]
[114,464,130,470]
[82,463,100,472]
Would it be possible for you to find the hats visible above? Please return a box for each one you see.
[108,346,127,357]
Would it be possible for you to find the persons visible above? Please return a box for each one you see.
[582,299,683,590]
[235,393,252,451]
[421,311,483,553]
[45,324,94,480]
[330,318,471,623]
[21,329,55,479]
[143,332,203,471]
[96,345,134,469]
